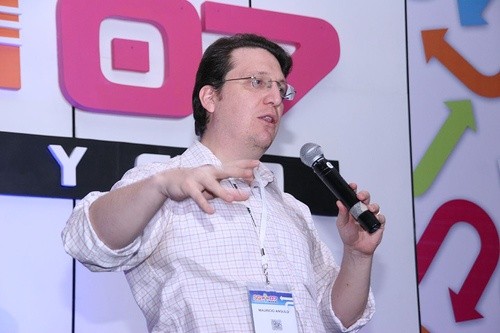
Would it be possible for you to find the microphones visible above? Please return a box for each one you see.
[299,142,381,234]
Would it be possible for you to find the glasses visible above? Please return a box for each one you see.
[211,73,296,101]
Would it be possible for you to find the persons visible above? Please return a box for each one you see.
[59,31,387,333]
